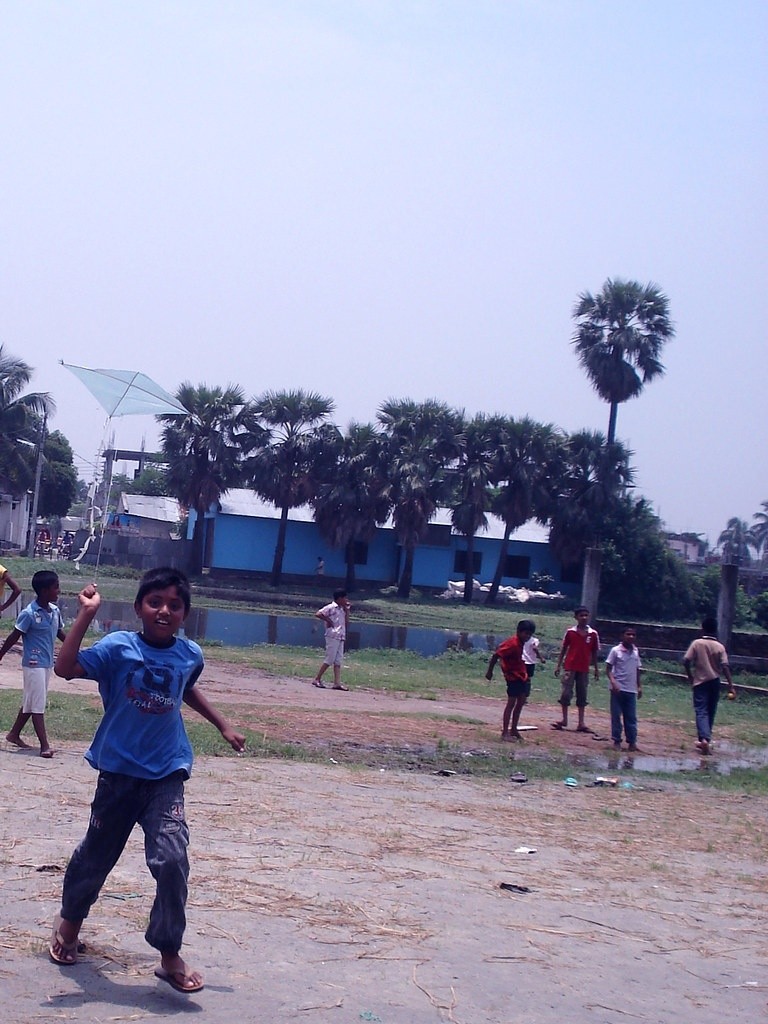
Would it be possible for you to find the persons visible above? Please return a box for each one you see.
[682,618,736,754]
[550,606,601,734]
[49,569,245,992]
[484,620,546,743]
[0,565,22,619]
[315,557,324,587]
[0,570,67,757]
[36,528,71,557]
[605,624,642,751]
[312,589,351,691]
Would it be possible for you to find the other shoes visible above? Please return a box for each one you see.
[628,744,641,752]
[576,726,594,733]
[613,742,627,752]
[700,739,710,755]
[510,731,524,740]
[551,721,565,730]
[501,733,516,742]
[695,741,711,748]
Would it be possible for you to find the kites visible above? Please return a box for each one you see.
[60,362,191,561]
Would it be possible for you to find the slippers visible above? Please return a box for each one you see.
[332,685,349,691]
[50,914,77,964]
[154,965,204,991]
[312,681,325,688]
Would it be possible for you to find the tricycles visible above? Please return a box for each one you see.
[57,528,78,561]
[33,528,53,559]
